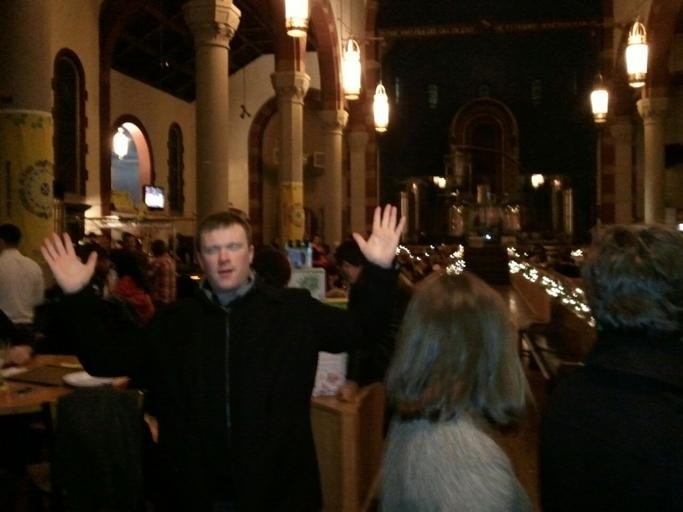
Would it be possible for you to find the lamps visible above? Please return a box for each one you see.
[336,38,390,137]
[283,0,309,38]
[589,14,649,124]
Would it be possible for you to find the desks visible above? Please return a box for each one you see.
[0,354,131,417]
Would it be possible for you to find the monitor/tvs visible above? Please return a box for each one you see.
[143,184,165,211]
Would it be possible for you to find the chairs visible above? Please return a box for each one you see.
[41,387,144,511]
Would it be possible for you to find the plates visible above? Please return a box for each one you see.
[61,370,113,387]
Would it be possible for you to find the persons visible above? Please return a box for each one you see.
[311,232,329,259]
[268,235,280,249]
[476,224,514,312]
[74,229,181,332]
[377,268,538,512]
[534,219,682,512]
[40,198,407,511]
[333,238,415,443]
[0,221,46,345]
